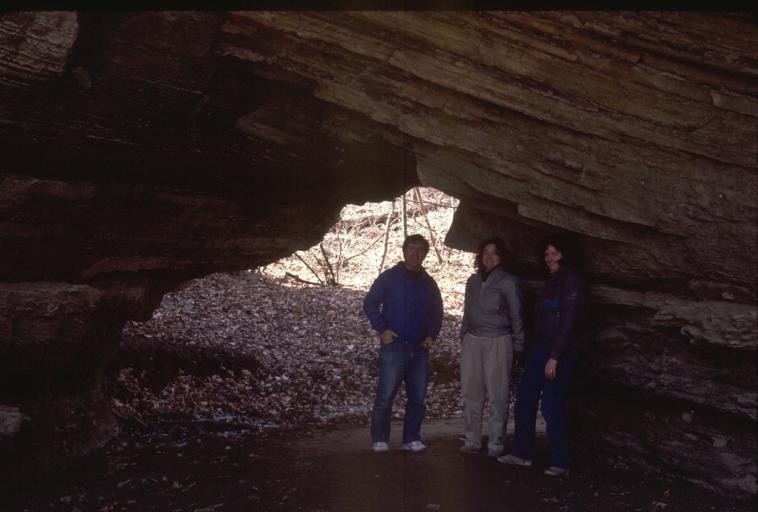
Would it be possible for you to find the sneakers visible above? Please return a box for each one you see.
[399,440,425,452]
[543,464,572,478]
[487,448,502,460]
[458,445,481,456]
[496,453,535,467]
[371,441,391,453]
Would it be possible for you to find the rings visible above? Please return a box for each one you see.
[423,342,428,346]
[549,369,552,372]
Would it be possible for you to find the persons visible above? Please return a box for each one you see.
[458,234,526,458]
[363,235,444,453]
[496,228,591,481]
